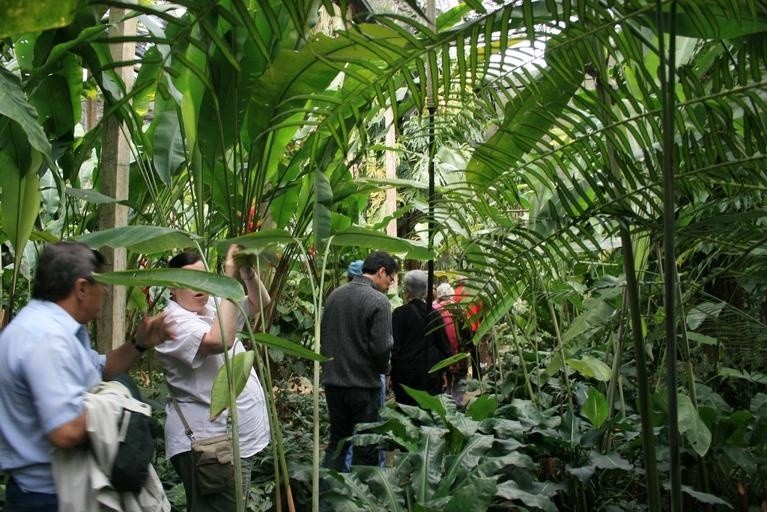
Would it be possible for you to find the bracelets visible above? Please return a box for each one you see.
[132,336,146,353]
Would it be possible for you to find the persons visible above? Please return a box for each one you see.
[151,244,272,512]
[0,241,178,512]
[321,250,491,472]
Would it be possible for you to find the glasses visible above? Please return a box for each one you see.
[378,263,395,284]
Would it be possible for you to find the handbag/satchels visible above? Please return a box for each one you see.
[191,432,236,499]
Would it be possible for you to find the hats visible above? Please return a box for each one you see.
[347,259,364,278]
